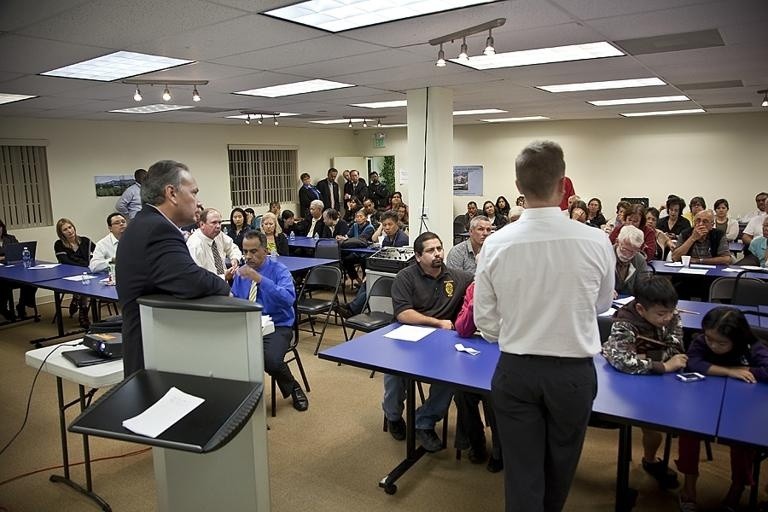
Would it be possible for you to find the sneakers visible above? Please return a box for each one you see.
[353,281,362,289]
[385,415,408,441]
[78,315,90,327]
[718,496,740,512]
[69,300,78,317]
[415,424,443,452]
[677,486,697,512]
[641,452,679,484]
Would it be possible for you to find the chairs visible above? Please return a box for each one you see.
[268,290,311,417]
[296,264,349,357]
[708,277,767,306]
[306,241,348,307]
[337,276,398,379]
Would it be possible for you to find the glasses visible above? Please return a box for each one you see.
[110,220,127,226]
[620,242,638,255]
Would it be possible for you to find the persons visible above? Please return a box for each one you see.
[53,217,95,329]
[382,177,768,510]
[115,160,234,378]
[0,219,28,322]
[473,141,616,511]
[90,166,410,412]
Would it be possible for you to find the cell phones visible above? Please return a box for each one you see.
[675,372,707,382]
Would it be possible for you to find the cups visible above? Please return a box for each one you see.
[682,255,691,267]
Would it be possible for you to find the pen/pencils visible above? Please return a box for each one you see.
[677,309,701,315]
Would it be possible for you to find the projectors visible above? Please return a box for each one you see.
[82,332,122,357]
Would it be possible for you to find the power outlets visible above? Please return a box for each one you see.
[418,207,429,220]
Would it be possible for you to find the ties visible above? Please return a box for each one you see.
[211,240,224,275]
[248,279,257,302]
[329,182,334,209]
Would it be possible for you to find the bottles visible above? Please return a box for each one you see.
[290,231,295,241]
[81,271,90,285]
[271,246,277,257]
[23,247,31,268]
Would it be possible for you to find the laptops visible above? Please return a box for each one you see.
[0,240,37,265]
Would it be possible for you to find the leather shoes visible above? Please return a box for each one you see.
[333,303,354,319]
[290,380,309,411]
[8,308,16,323]
[467,435,488,465]
[16,303,27,319]
[486,444,508,473]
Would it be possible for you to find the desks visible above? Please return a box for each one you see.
[319,321,729,512]
[33,271,118,337]
[266,254,342,328]
[285,236,381,257]
[715,377,767,512]
[727,242,746,258]
[0,259,102,338]
[596,293,768,336]
[26,337,153,512]
[648,257,768,302]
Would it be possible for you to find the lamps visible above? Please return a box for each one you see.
[760,92,768,108]
[239,109,280,126]
[122,78,209,103]
[429,18,506,66]
[342,115,386,129]
